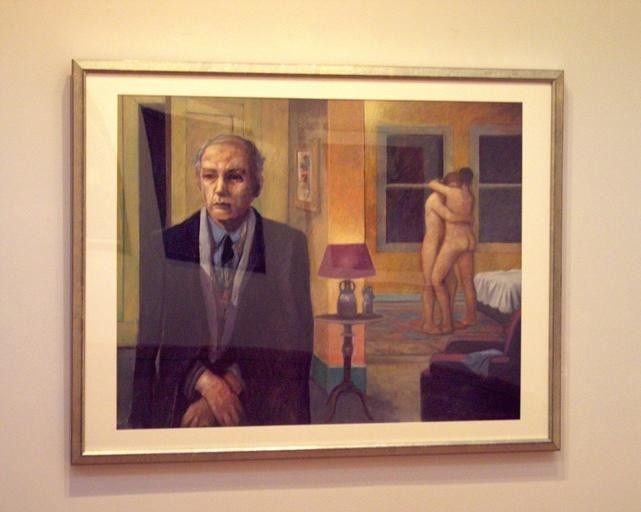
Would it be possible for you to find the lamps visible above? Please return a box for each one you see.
[318,244,378,317]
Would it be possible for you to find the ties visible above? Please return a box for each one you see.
[222,234,234,305]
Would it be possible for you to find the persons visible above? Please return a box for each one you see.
[420,171,476,335]
[427,167,476,334]
[129,135,314,428]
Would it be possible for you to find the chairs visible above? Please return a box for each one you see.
[420,311,521,422]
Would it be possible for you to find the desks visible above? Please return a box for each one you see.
[313,312,384,423]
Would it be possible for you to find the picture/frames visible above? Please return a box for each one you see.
[291,139,321,216]
[68,55,565,470]
